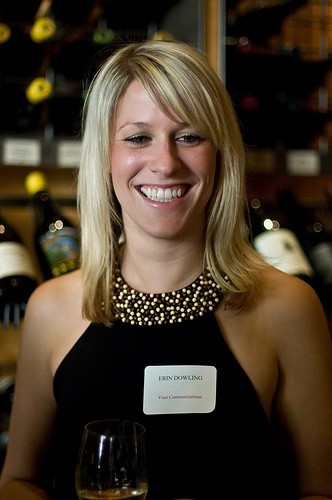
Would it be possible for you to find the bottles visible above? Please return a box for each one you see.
[1,1,331,473]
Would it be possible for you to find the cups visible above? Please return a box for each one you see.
[76,420,149,500]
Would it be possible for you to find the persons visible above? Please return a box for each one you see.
[0,38,332,500]
